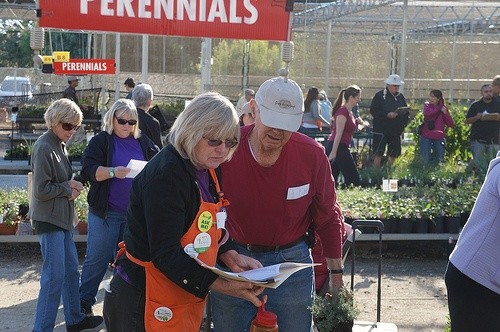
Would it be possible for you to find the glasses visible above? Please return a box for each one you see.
[114,115,137,125]
[202,136,238,148]
[59,121,80,131]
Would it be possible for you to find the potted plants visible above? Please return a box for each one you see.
[0,206,18,235]
[307,281,361,332]
[76,201,89,235]
[336,183,481,235]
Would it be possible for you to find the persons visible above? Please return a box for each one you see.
[420,90,454,163]
[132,83,163,149]
[492,75,500,96]
[318,90,359,146]
[466,84,500,157]
[211,77,352,332]
[81,98,160,316]
[236,88,255,127]
[301,86,331,131]
[443,150,500,332]
[326,85,361,188]
[124,78,135,100]
[30,98,103,332]
[62,77,87,111]
[370,74,408,166]
[102,91,268,332]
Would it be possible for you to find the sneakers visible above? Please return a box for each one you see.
[80,301,94,317]
[66,316,104,332]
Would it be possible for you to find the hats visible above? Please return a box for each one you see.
[384,75,404,85]
[255,76,305,132]
[68,76,80,82]
[242,102,251,114]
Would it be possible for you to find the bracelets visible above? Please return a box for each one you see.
[110,167,115,178]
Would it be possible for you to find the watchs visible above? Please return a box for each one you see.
[328,264,345,274]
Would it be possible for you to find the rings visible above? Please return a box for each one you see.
[254,289,256,295]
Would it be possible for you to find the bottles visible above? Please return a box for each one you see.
[250,301,278,332]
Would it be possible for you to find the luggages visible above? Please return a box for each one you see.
[313,220,397,332]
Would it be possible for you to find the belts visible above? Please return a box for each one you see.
[233,236,305,253]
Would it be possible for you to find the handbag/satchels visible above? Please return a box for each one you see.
[416,124,424,135]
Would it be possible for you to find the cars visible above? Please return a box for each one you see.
[0,75,34,108]
